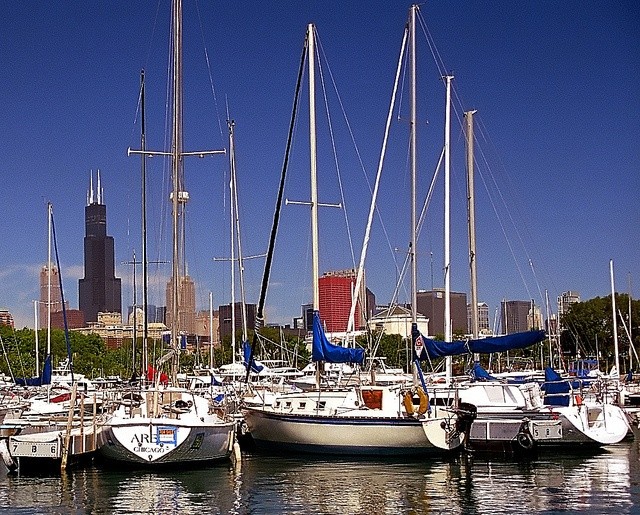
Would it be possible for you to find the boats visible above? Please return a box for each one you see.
[0,418,100,471]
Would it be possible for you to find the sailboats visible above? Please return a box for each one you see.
[2,200,90,414]
[244,1,477,456]
[343,2,569,397]
[577,260,639,429]
[364,109,569,380]
[369,77,630,449]
[100,0,245,466]
